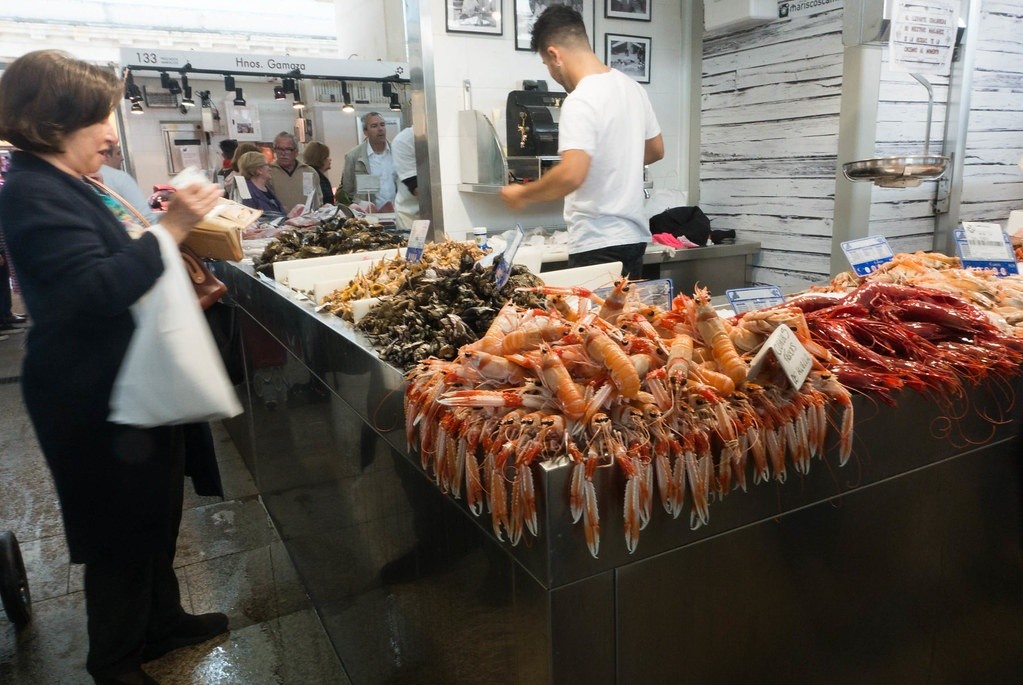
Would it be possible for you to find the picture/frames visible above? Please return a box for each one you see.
[514,0,596,54]
[605,33,652,84]
[445,0,503,36]
[604,0,652,22]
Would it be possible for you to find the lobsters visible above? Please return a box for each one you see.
[724,250,1023,450]
[401,274,855,559]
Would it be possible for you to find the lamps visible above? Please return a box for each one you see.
[119,63,412,115]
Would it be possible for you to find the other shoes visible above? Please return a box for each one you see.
[87,662,160,685]
[1,312,32,342]
[377,544,449,585]
[135,605,229,664]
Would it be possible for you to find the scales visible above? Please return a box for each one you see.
[842,0,960,188]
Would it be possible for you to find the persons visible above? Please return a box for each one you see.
[0,51,229,685]
[334,111,396,215]
[0,131,322,334]
[499,5,665,285]
[392,127,418,238]
[302,142,334,206]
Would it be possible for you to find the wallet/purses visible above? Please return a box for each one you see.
[179,197,265,262]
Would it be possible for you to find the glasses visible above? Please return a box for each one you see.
[274,147,296,155]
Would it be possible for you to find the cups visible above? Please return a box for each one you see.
[473,227,487,250]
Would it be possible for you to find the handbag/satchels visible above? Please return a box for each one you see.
[105,225,245,428]
[85,176,228,313]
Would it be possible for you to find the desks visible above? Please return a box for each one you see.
[459,236,762,300]
